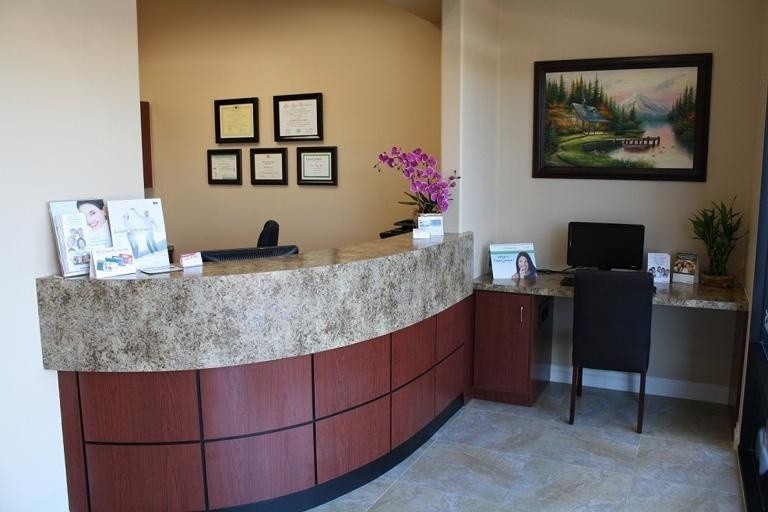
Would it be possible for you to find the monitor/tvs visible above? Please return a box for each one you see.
[199,244,300,260]
[565,219,645,272]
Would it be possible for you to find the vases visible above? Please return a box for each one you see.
[420,213,443,217]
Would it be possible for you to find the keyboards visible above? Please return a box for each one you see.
[558,274,658,293]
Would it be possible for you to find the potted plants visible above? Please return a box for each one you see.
[688,195,751,290]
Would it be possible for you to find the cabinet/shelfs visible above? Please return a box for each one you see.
[473,291,555,408]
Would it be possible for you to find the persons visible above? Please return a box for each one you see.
[131,207,160,254]
[511,252,536,280]
[76,199,107,231]
[674,261,696,274]
[122,208,139,258]
[649,265,669,279]
[67,226,86,254]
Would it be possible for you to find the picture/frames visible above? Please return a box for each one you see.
[531,53,713,183]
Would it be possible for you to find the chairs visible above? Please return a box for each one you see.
[569,269,654,434]
[256,220,279,248]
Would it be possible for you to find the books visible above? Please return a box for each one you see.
[48,199,112,278]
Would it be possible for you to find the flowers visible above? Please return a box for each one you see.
[372,146,461,214]
